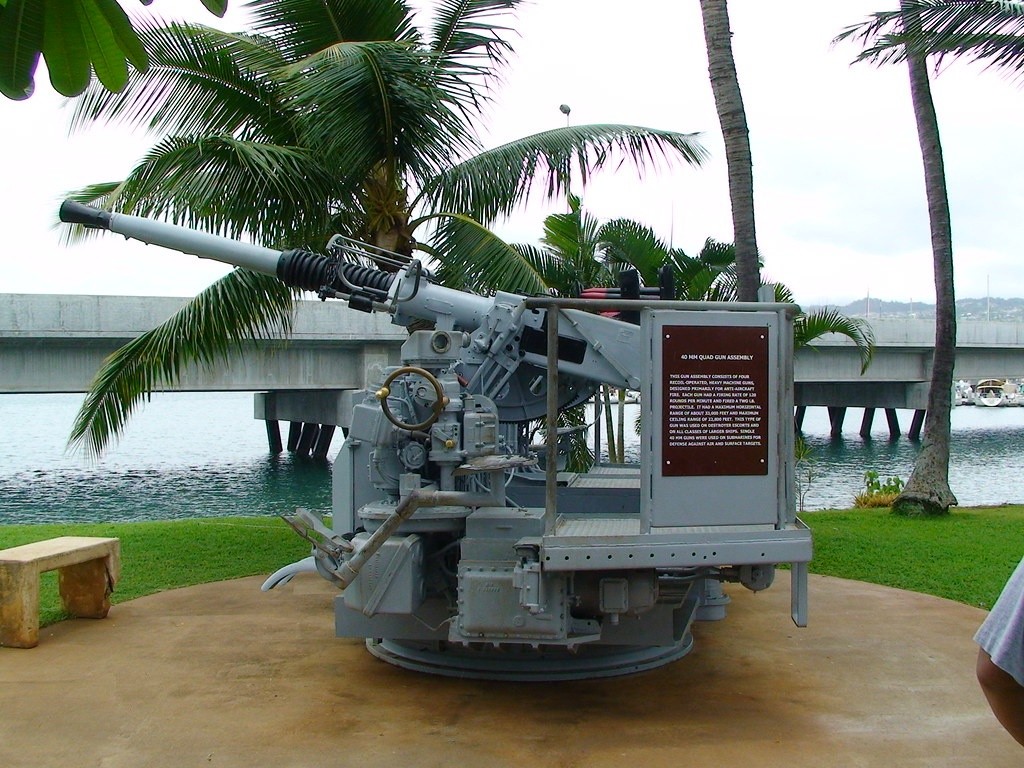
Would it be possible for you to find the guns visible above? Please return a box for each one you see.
[56,196,816,396]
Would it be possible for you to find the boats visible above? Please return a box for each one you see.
[955,377,1024,408]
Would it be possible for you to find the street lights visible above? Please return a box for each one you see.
[559,104,570,215]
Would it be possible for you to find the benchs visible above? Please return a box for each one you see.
[0,536,121,648]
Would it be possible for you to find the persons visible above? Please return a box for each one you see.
[974,555,1024,748]
